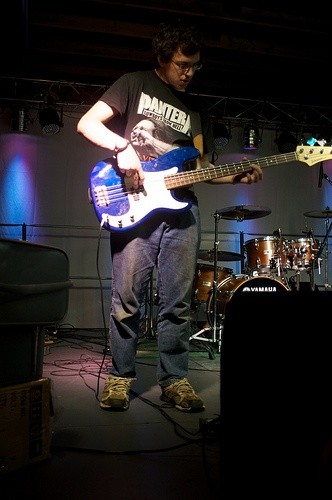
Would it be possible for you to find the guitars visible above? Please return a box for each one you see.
[89,137,331,232]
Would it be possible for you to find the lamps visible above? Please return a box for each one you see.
[241,122,260,150]
[38,102,65,137]
[272,128,298,155]
[8,103,34,135]
[212,118,233,148]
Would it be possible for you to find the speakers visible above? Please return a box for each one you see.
[220,290,332,500]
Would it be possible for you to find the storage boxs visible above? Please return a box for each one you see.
[0,377,55,475]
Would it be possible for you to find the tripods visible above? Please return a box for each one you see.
[186,214,245,343]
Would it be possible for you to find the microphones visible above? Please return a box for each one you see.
[273,228,280,236]
[318,162,323,188]
[307,228,312,237]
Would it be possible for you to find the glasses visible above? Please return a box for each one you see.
[170,57,203,71]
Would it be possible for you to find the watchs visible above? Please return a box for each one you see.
[113,139,130,158]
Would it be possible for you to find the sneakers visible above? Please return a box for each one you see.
[100,373,136,408]
[161,377,205,412]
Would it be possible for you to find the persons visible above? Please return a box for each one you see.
[77,26,262,412]
[131,116,178,160]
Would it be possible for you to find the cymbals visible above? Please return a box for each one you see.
[197,249,246,262]
[213,206,271,220]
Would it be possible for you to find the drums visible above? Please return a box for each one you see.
[195,263,234,306]
[282,238,320,271]
[206,274,290,331]
[241,236,290,274]
